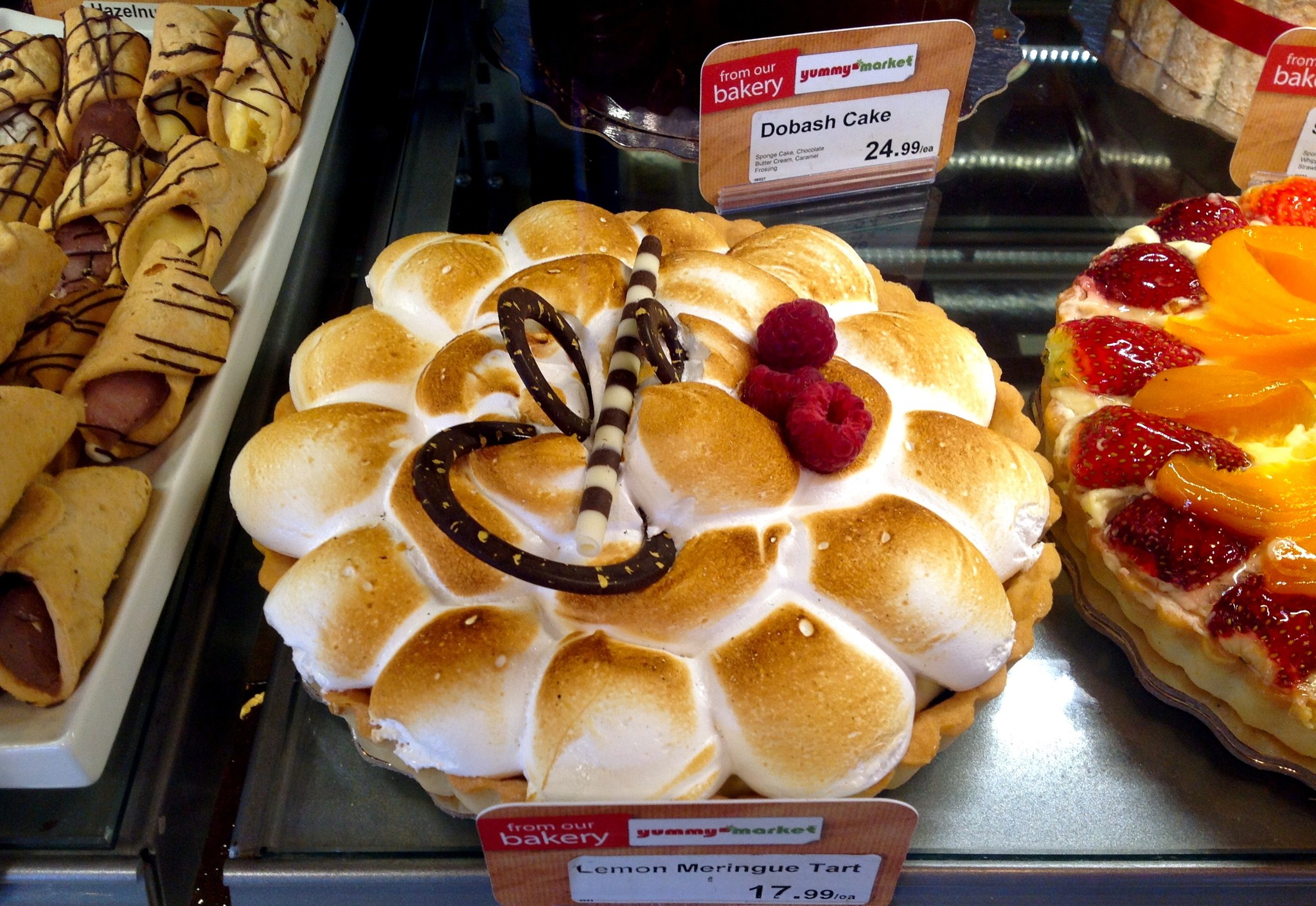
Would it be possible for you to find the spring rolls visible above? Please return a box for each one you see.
[0,0,332,705]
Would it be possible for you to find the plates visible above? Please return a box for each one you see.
[0,14,358,791]
[301,678,478,822]
[1031,384,1316,792]
[487,0,1026,165]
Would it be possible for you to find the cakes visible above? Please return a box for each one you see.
[230,196,1065,821]
[1038,174,1315,771]
